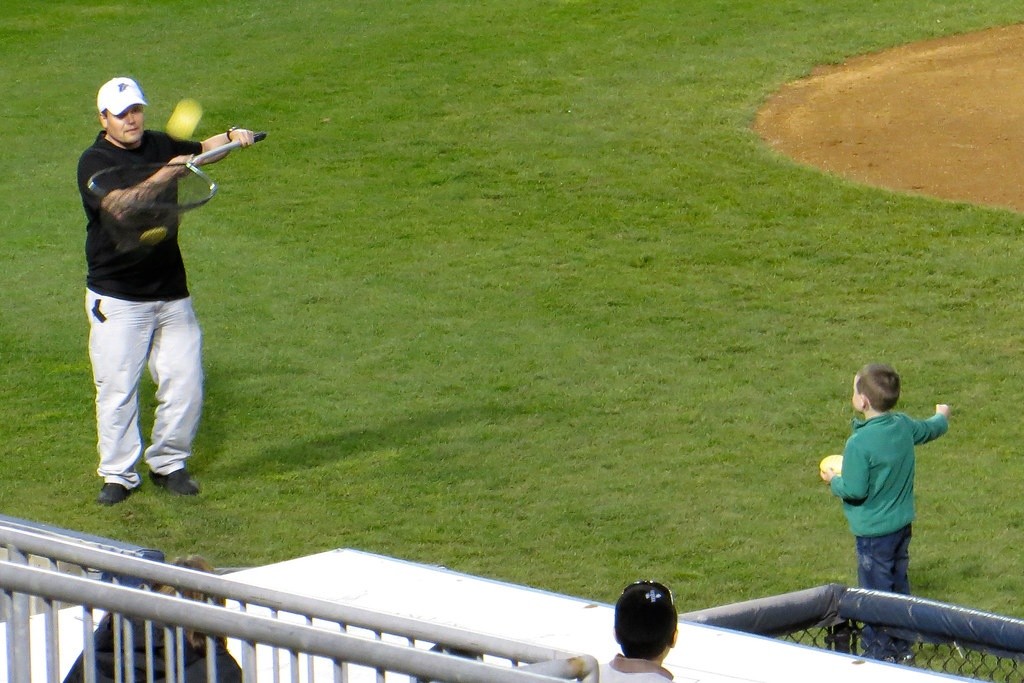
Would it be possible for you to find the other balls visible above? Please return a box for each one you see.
[820,454,842,482]
[165,98,203,142]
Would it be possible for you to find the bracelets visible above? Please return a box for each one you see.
[227,126,237,142]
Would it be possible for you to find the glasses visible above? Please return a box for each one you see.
[622,580,675,602]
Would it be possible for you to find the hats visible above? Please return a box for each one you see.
[96,78,149,115]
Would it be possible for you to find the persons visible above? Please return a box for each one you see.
[75,77,255,504]
[819,361,950,666]
[598,581,680,683]
[63,548,243,683]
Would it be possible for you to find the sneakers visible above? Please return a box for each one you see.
[92,476,143,508]
[150,466,202,495]
